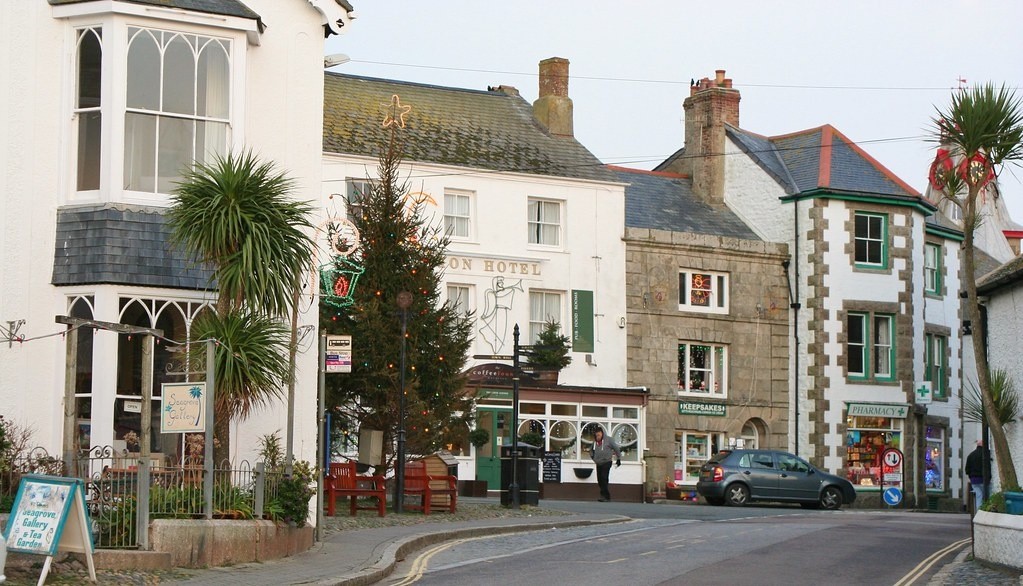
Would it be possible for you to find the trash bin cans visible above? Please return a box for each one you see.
[501,441,539,507]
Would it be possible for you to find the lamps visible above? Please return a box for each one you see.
[324,53,350,69]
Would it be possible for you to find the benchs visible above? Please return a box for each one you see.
[394,459,458,515]
[325,461,387,517]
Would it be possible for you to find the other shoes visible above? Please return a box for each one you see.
[598,496,610,502]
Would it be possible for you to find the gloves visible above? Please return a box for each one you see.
[616,459,621,466]
[591,456,594,459]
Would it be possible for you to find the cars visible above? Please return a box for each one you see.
[696,447,856,509]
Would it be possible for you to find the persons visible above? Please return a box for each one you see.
[589,426,621,502]
[964,439,992,509]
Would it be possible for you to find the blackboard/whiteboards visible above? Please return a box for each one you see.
[3,472,77,555]
[542,451,561,483]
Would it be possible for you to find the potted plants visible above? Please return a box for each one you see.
[464,427,488,497]
